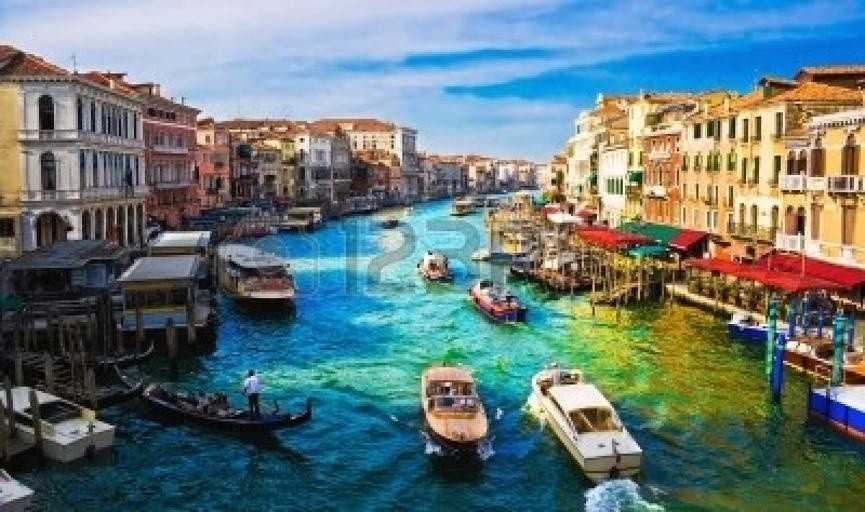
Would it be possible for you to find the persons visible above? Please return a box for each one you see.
[240,366,263,420]
[188,386,229,418]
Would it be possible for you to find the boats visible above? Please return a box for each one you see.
[416,248,454,283]
[420,359,490,445]
[531,361,644,487]
[728,311,768,345]
[380,219,399,230]
[472,193,576,279]
[467,277,529,325]
[450,194,501,216]
